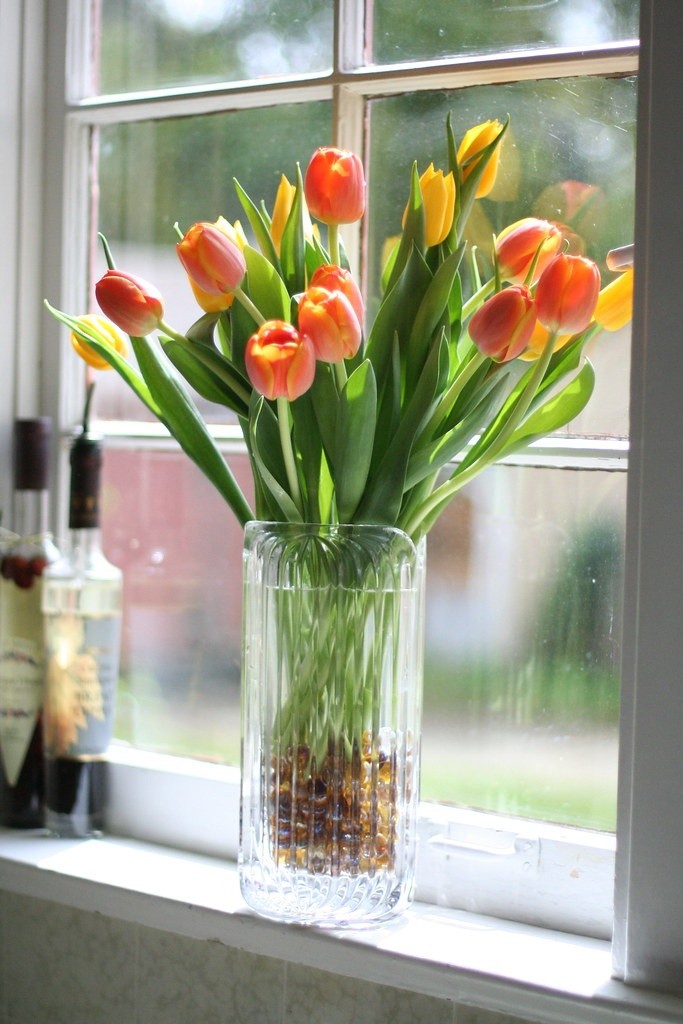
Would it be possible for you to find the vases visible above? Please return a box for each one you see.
[236,517,428,928]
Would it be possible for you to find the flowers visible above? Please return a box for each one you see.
[37,114,634,541]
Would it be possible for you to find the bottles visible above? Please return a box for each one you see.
[0,416,65,830]
[41,434,123,838]
